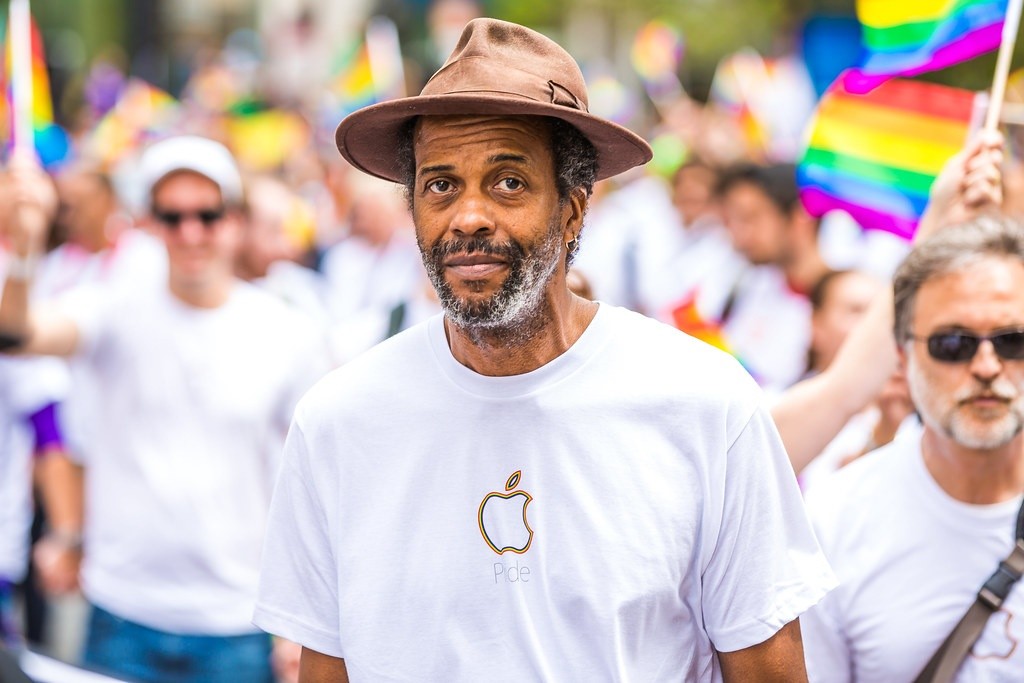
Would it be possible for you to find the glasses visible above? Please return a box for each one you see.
[152,211,223,226]
[905,326,1024,363]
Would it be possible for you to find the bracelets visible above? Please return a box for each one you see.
[8,256,35,281]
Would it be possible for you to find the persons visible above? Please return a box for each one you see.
[0,135,349,683]
[0,104,1024,646]
[798,216,1024,683]
[251,17,840,683]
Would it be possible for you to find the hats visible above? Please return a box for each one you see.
[131,136,242,214]
[336,19,654,179]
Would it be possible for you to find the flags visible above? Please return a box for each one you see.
[800,68,979,244]
[0,15,54,152]
[854,0,1008,79]
